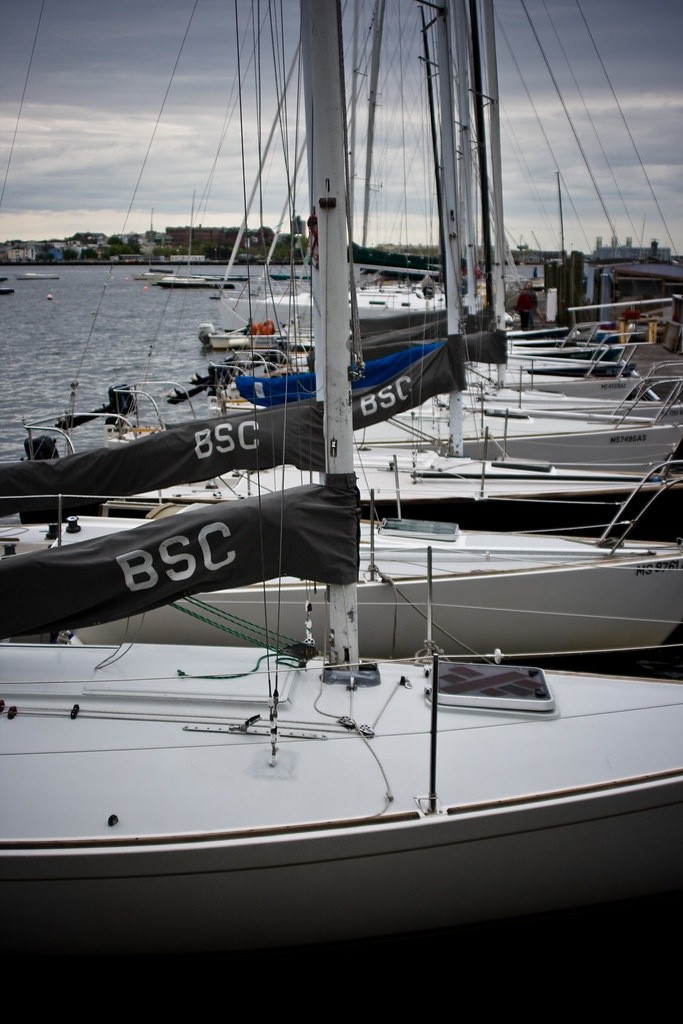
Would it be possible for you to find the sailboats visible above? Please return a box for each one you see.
[0,0,683,1024]
